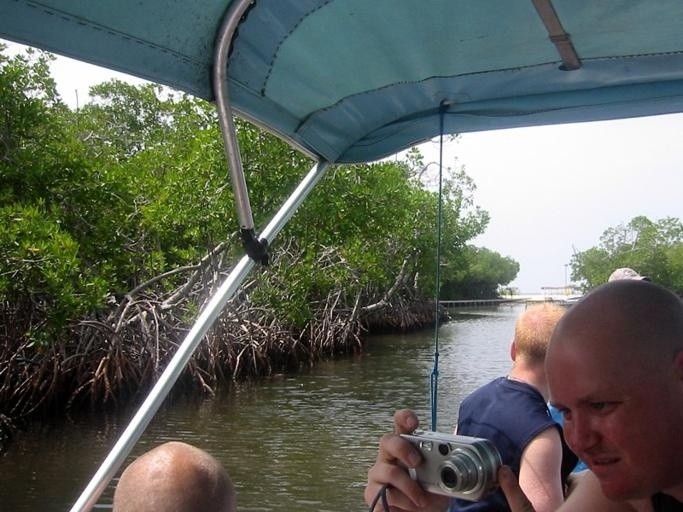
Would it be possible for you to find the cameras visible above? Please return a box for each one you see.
[401,431,502,502]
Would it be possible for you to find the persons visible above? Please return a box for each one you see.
[364,280,683,512]
[111,441,237,512]
[448,268,648,512]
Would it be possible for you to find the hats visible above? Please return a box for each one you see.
[608,268,651,283]
